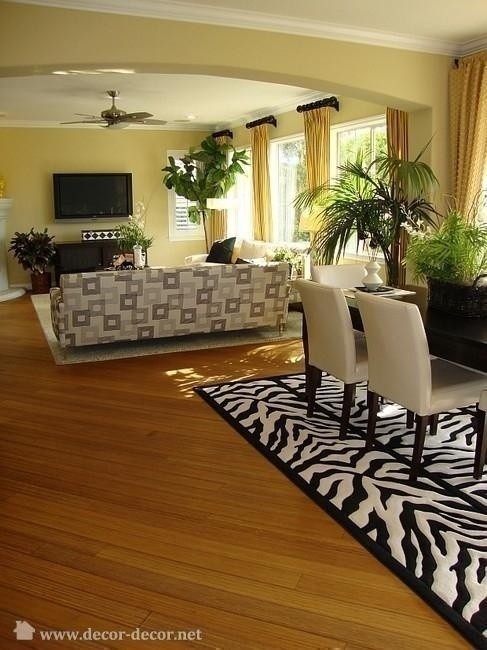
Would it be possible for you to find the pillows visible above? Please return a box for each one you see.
[206,236,236,262]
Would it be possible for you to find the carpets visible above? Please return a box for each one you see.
[30,293,309,366]
[195,371,486,649]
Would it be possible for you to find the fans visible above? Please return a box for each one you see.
[59,91,167,129]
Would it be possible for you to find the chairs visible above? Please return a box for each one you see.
[357,289,487,485]
[293,277,370,442]
[309,264,370,289]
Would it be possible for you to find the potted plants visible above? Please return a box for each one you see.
[7,227,56,294]
[112,221,157,268]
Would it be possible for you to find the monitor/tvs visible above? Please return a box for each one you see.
[53,173,133,224]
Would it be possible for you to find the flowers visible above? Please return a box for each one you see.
[358,228,384,261]
[395,195,486,282]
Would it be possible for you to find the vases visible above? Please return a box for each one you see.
[361,262,384,292]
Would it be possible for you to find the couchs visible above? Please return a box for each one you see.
[185,236,310,304]
[47,258,290,359]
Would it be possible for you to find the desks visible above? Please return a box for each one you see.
[288,284,487,414]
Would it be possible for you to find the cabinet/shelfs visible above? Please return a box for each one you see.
[425,283,487,372]
[54,240,147,287]
